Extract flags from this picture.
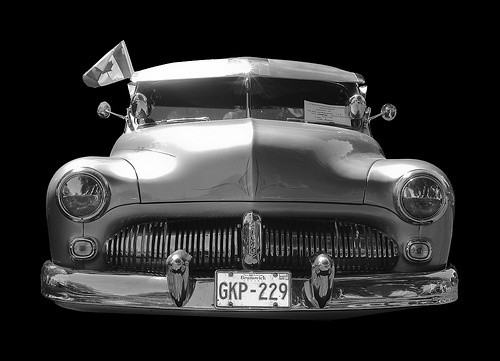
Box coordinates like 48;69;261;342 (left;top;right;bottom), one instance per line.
82;40;135;88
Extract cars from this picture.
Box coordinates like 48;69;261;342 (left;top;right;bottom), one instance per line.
43;54;460;322
